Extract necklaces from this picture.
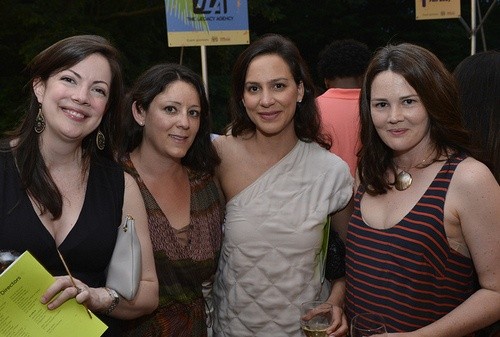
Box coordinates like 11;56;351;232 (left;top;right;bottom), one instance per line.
389;143;437;191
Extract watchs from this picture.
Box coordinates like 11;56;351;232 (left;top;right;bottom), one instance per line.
99;286;119;316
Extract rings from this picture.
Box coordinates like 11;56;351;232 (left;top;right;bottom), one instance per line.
72;285;80;297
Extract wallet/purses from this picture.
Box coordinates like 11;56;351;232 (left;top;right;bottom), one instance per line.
325;230;346;282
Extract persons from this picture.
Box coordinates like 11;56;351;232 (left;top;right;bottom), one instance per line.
202;32;355;337
0;35;161;337
342;42;500;337
452;50;500;187
114;63;226;337
309;39;371;188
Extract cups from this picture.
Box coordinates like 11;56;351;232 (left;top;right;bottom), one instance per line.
350;313;387;337
301;302;333;337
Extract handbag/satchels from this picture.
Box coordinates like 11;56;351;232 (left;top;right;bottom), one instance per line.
106;216;142;301
